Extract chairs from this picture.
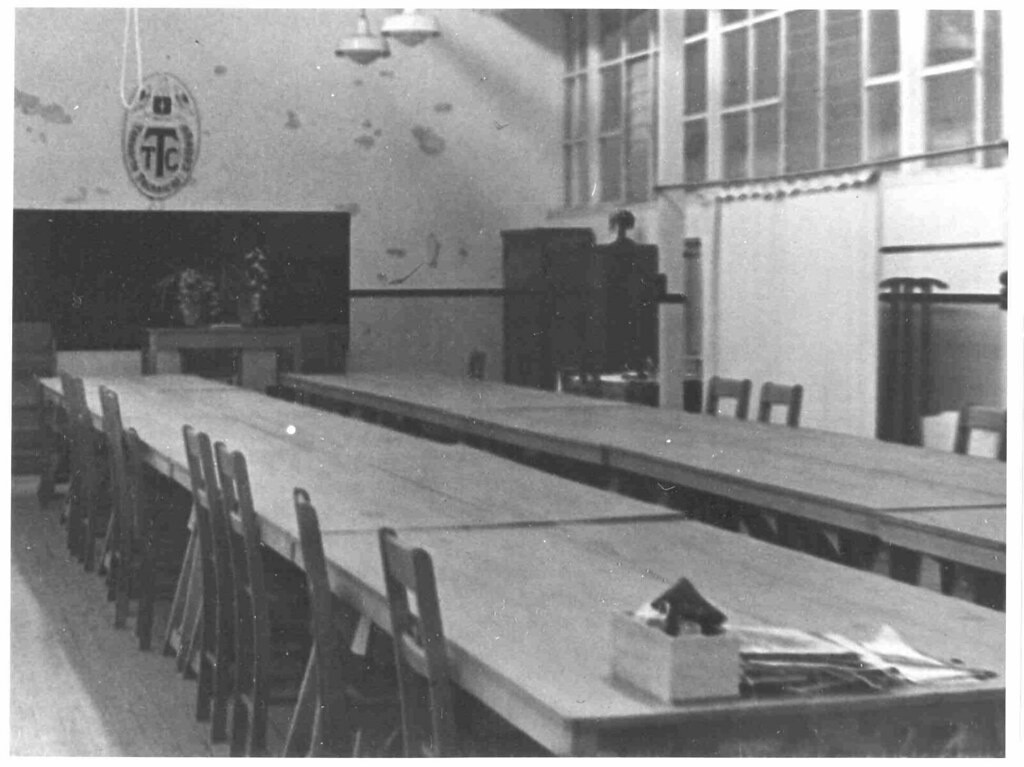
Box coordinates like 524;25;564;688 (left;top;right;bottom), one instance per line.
37;367;555;758
572;373;1007;462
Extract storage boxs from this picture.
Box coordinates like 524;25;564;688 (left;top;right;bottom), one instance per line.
609;609;741;709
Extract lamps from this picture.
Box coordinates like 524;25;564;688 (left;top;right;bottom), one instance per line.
334;9;442;65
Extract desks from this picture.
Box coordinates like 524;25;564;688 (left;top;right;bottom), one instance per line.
38;373;245;434
80;391;450;578
292;517;1006;758
444;407;735;469
604;423;1007;545
278;370;649;431
878;504;1006;595
161;442;684;678
